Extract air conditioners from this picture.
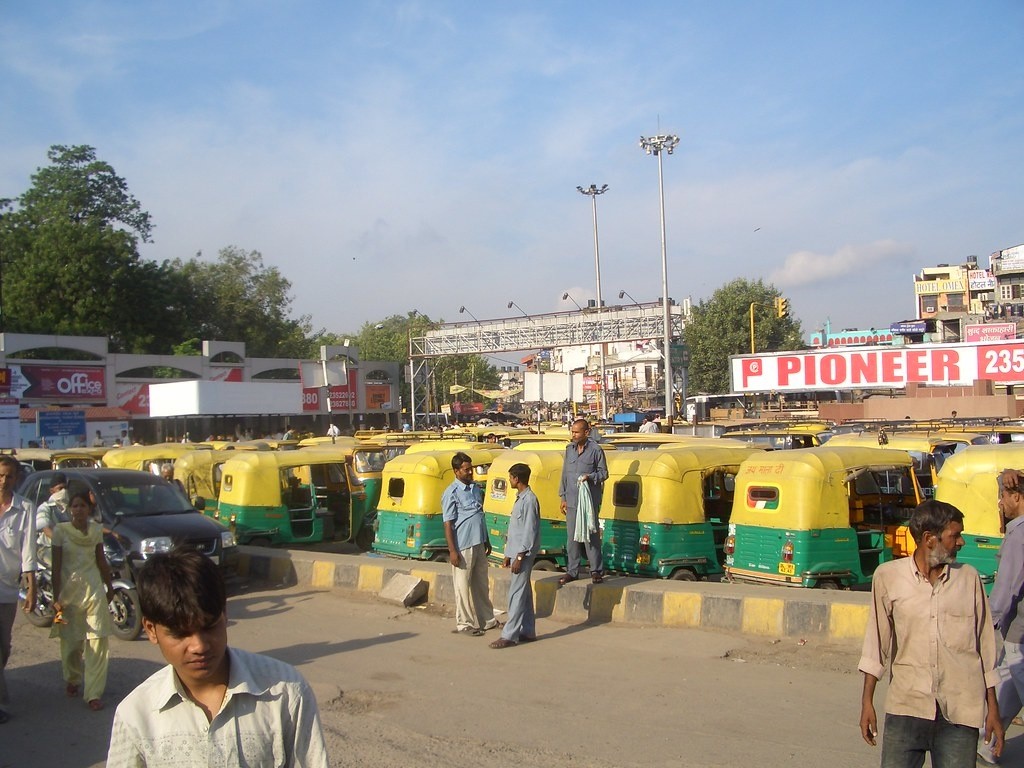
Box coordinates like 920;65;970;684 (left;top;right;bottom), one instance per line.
926;306;935;313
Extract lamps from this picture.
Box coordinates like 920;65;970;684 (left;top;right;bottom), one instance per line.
563;293;589;317
460;307;484;328
508;301;535;324
619;290;643;310
411;309;437;331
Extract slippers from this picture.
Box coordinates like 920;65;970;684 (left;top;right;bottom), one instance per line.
519;634;537;642
491;620;506;628
489;638;514;649
459;628;485;635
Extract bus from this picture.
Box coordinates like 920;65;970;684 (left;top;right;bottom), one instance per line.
678;388;855;422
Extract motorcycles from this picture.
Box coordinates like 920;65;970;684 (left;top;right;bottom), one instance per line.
16;512;144;641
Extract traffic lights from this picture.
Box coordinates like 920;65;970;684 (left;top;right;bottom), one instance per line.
779;298;789;318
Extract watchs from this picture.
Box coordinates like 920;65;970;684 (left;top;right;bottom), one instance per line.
516;554;523;561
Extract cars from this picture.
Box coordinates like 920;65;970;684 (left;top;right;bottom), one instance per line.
16;468;243;596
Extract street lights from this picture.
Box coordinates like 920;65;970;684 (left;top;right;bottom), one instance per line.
639;135;681;416
576;183;610;419
344;339;354;437
318;360;336;444
590;349;652;399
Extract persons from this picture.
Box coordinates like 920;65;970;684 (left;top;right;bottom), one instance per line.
139;462;191;507
441;451;505;636
25;441;48;470
637;416;659;451
370;417;575;434
73;429;144;469
0;472;115;711
559;420;609;584
950;411;957;424
858;499;1006;768
182;423;340;443
104;552;331;768
979;469;1024;767
488;463;540;650
477;433;511;473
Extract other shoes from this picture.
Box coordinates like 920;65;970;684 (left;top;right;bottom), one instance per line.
560;574;579;584
90;699;105;710
592;573;603;583
67;682;79;698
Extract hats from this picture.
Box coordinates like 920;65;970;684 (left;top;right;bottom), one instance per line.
50;472;66;488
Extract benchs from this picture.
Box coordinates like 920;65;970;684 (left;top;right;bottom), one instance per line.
856;529;893;571
288;488;336;518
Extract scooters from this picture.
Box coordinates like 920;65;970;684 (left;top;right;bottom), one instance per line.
483;450;591;572
371;449;506;565
0;441;287;522
596;425;749;451
215;450;367;545
294;445;387;525
934;440;1024;597
720;446;926;589
719;417;1024;499
585;446;767;581
258;427;573;460
